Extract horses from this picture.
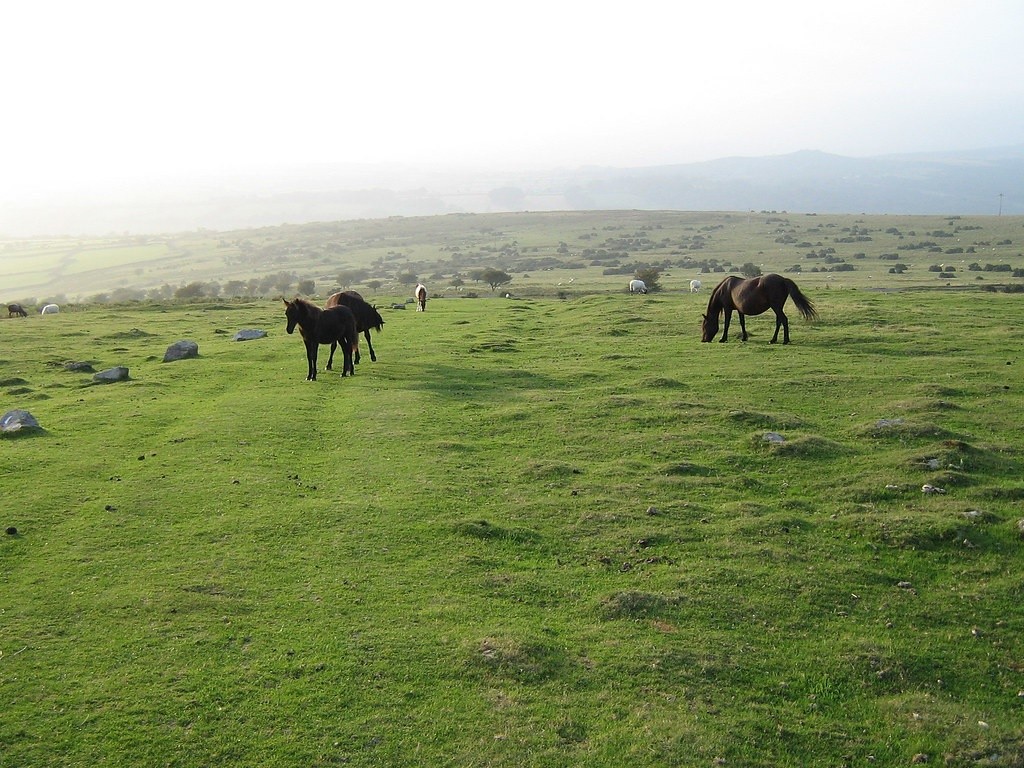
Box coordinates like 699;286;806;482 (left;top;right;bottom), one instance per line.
690;280;702;293
8;304;27;317
324;290;385;373
40;304;59;315
282;297;358;382
629;279;648;295
414;283;428;312
701;273;821;345
